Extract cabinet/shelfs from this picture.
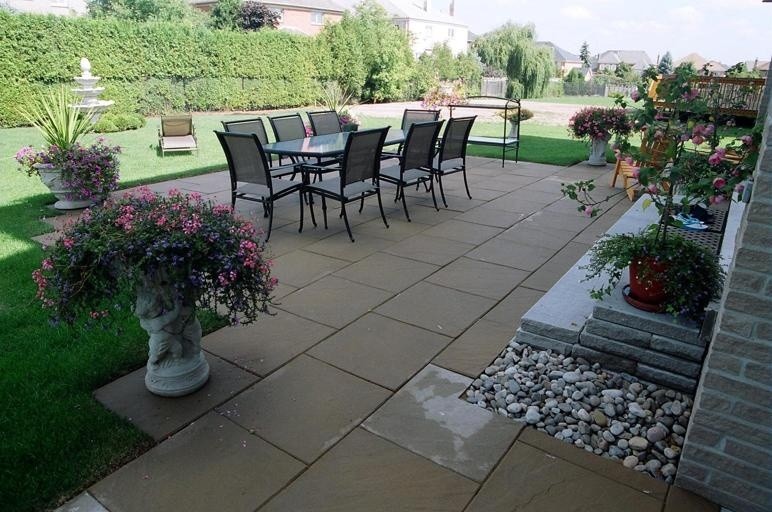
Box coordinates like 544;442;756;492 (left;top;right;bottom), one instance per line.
437;95;522;169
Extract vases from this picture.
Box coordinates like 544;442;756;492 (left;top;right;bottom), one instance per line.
112;258;214;401
583;130;608;169
31;159;107;211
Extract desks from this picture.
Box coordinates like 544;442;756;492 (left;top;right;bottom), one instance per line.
262;127;411;229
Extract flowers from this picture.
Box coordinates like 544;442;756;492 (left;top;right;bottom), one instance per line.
13;137;127;211
29;182;289;350
565;104;632;145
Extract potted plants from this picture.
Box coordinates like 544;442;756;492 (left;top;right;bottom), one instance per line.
574;217;728;323
499;104;536;149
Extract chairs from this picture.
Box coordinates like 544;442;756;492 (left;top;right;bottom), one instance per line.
605;129;682;203
154;114;200;159
220;111;351;206
421;115;478;209
359;119;444;222
306;125;391;243
212;130;307;242
